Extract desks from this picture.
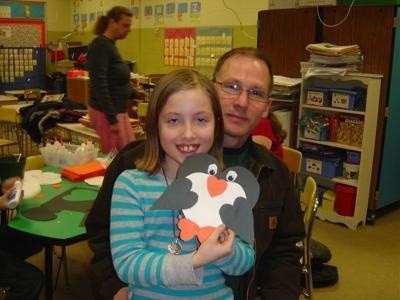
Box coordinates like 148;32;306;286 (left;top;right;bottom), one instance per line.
4;90;48;100
0;95;18;105
7;153;115;300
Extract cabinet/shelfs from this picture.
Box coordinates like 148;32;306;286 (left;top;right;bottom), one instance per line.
296;72;383;231
258;6;322;77
322;7;389;223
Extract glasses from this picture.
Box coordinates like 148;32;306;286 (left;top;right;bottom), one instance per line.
215;79;269;104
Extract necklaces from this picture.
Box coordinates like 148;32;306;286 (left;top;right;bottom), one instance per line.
162;167;182;255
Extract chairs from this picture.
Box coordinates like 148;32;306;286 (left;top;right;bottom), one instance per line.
299;175;316;300
23;154;44;177
278;148;301;188
137;103;148;132
0;108;23;156
25;91;40;104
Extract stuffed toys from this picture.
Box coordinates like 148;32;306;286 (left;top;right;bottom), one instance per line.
149;153;261;248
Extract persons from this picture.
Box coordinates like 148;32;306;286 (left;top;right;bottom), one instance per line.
0;176;47;300
86;7;140;152
110;69;255;300
86;46;308;300
